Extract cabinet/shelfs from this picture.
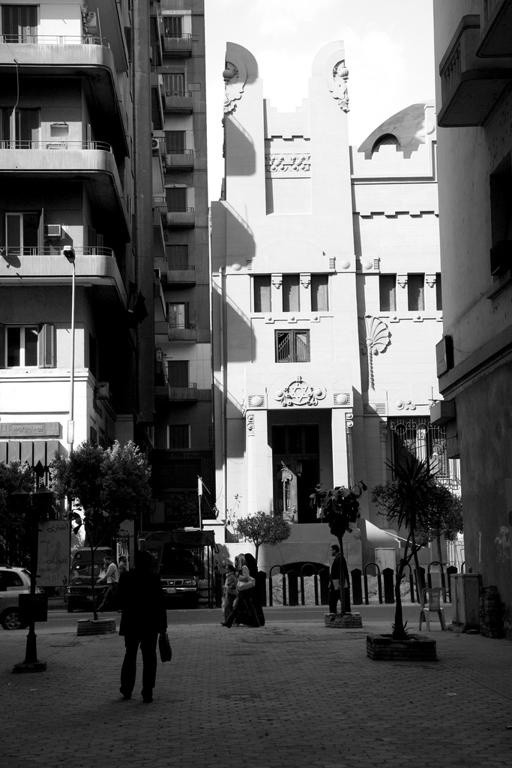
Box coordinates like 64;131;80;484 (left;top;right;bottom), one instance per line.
449;573;480;625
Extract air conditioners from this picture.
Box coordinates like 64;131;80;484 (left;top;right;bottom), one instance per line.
152;138;160;151
44;224;62;238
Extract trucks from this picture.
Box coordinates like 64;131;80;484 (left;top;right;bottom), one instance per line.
145;546;195;609
66;546;115;612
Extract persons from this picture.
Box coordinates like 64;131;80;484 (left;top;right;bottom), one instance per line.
214;554;267;627
118;548;167;704
328;544;349;620
96;554;119;612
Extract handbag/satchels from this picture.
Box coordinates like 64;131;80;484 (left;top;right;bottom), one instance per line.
159;633;172;662
237;575;255;591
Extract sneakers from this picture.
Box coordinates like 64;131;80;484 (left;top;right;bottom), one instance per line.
221;621;232;628
120;687;153;702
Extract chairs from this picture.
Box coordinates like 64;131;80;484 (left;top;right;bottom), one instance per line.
419;587;447;633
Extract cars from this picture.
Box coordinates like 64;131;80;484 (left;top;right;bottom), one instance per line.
0;564;47;629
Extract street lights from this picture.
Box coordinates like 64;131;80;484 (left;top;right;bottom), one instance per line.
62;245;77;602
